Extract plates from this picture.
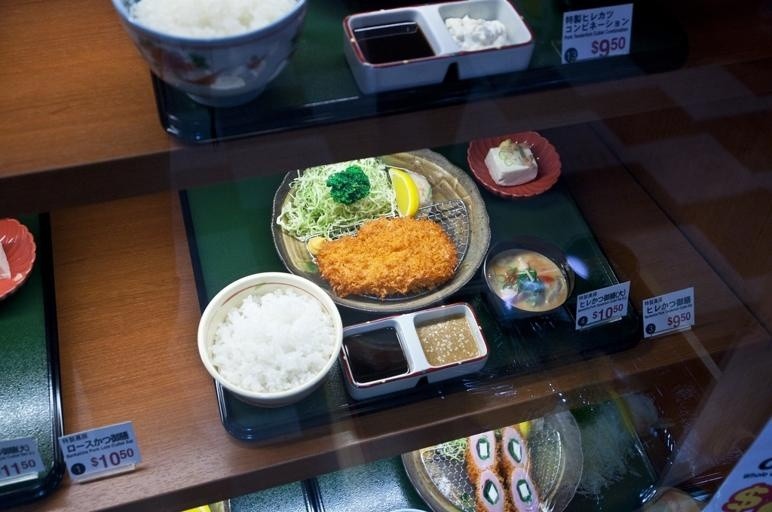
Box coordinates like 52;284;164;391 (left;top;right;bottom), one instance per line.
401;411;584;512
344;1;535;94
342;303;489;399
0;218;39;302
270;148;491;314
467;131;563;199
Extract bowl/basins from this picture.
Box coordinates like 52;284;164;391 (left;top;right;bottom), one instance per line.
114;0;307;109
482;234;576;318
197;271;342;409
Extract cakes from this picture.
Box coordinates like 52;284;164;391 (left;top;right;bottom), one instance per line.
485;138;542;188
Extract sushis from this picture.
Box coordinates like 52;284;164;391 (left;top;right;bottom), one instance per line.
464;426;541;512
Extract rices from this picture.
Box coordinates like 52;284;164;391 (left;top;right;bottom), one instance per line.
209;285;336;395
130;1;303;38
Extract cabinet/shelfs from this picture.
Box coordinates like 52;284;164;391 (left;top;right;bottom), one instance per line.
0;3;771;512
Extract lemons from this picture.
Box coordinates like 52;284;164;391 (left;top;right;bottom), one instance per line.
389;168;416;218
519;421;531;439
